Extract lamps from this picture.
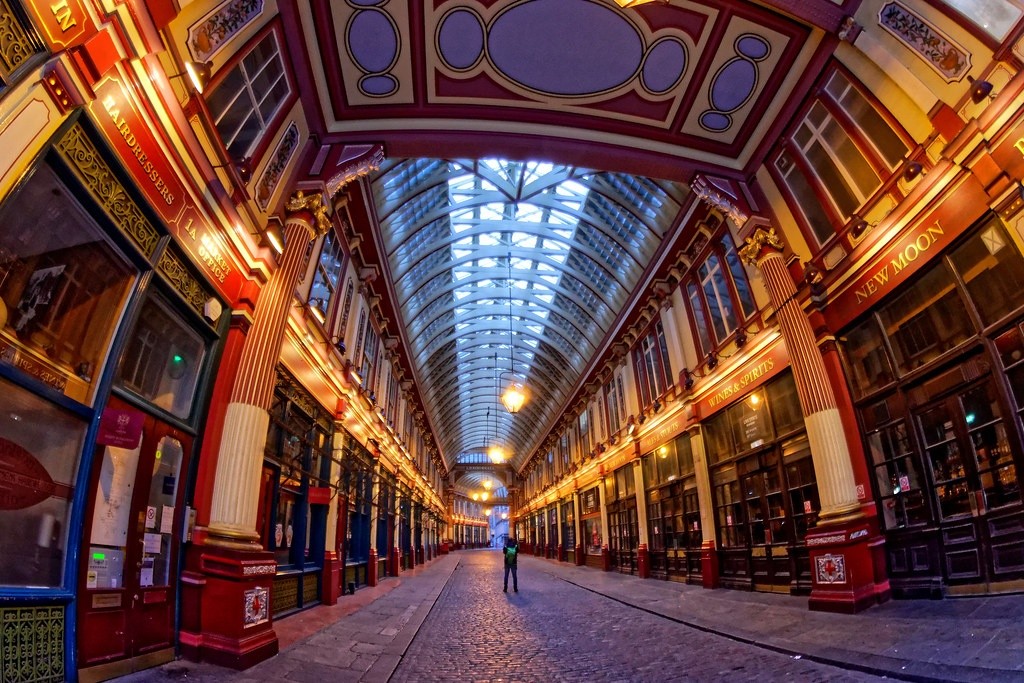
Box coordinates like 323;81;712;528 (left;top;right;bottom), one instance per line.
685;372;706;390
735;326;758;348
967;75;994;105
250;225;285;255
295;296;326;323
627;423;635;435
653;396;673;413
213;157;252;182
499;252;530;414
901;155;925;183
339;364;364;385
638;410;655;424
849;214;875;239
804;261;832;284
708;350;731;369
358;389;447;510
487;352;505;464
320;335;346;355
473;407;627;502
168;60;213;94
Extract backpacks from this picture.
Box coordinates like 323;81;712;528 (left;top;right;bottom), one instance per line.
504;544;518;564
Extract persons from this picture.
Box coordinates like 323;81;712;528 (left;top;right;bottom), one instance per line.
503;537;519;593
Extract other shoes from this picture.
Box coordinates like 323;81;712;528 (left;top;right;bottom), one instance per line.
514;588;518;592
503;590;507;592
